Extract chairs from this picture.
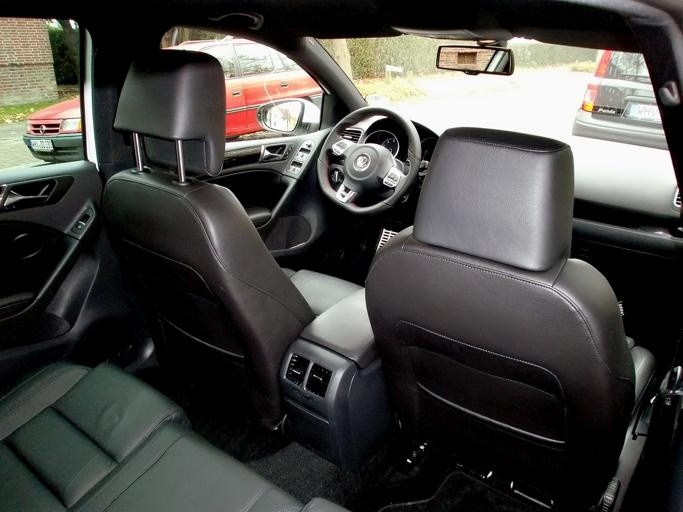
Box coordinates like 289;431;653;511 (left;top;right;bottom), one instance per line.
0;359;194;512
105;435;305;511
301;495;351;512
98;48;364;430
364;127;654;511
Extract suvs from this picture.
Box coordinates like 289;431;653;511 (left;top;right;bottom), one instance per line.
571;49;672;153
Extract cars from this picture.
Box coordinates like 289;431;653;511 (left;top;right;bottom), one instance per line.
19;36;326;163
